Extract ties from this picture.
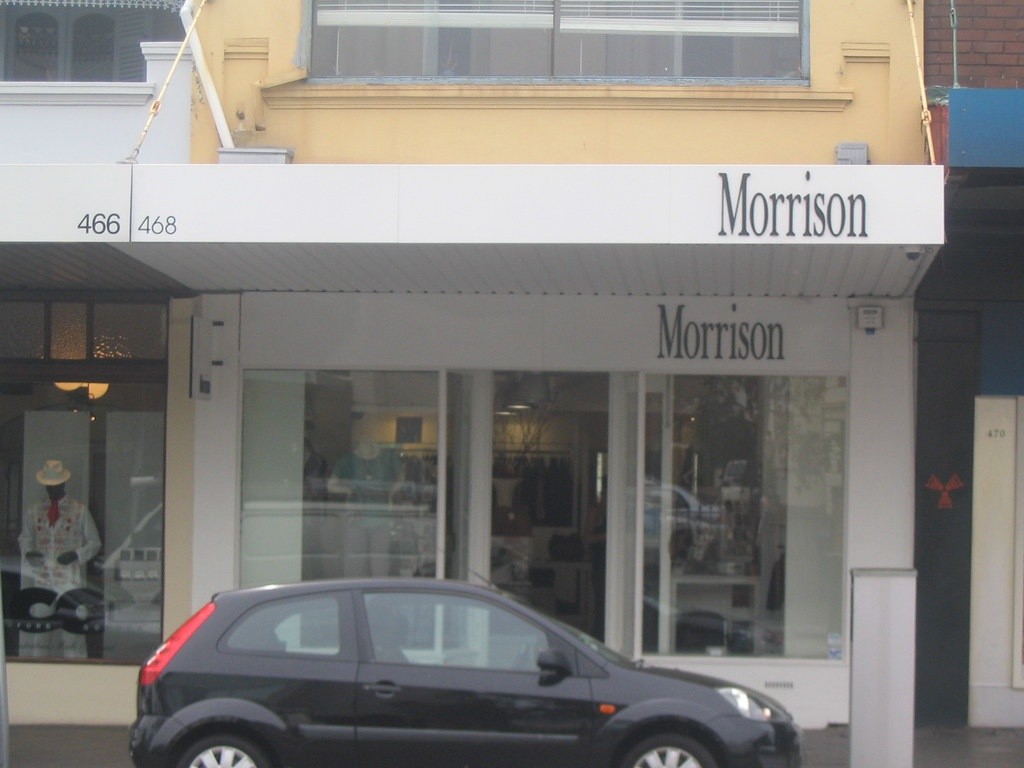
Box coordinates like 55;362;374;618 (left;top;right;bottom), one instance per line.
48;501;60;528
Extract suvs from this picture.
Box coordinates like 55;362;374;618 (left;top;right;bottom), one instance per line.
126;575;804;768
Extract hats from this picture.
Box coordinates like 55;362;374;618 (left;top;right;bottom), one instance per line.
37;460;70;485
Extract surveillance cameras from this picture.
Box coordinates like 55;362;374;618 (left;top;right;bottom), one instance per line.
904;247;921;261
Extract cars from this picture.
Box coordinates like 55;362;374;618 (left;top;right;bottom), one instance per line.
649;484;731;554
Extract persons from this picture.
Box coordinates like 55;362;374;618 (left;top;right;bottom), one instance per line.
19;459;102;658
325;435;407;580
584;474;608;642
